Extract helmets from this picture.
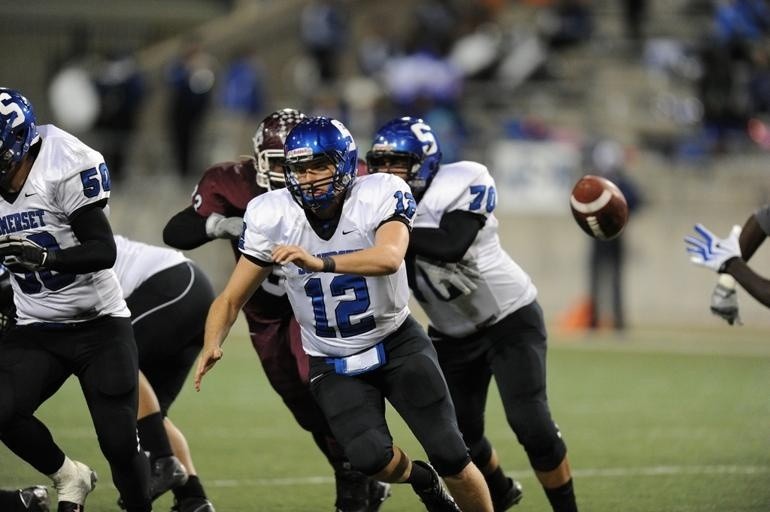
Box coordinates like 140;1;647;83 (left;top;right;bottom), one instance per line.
252;108;303;182
0;87;40;159
283;115;356;204
366;114;443;195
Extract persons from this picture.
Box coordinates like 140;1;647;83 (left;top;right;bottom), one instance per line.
0;85;152;511
111;233;216;511
366;116;579;511
1;1;770;215
585;146;647;329
682;207;770;323
1;485;52;512
164;108;393;511
194;115;496;511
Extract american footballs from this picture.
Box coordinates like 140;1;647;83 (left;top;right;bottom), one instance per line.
570;173;630;241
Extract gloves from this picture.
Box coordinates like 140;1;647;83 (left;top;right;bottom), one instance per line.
206;212;245;238
686;224;741;272
710;277;741;325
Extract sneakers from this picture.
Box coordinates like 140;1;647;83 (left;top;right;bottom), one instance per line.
116;458;216;512
493;477;523;510
0;460;97;512
364;480;391;512
411;455;457;512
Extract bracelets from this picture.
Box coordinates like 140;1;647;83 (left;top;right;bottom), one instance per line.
316;255;336;273
